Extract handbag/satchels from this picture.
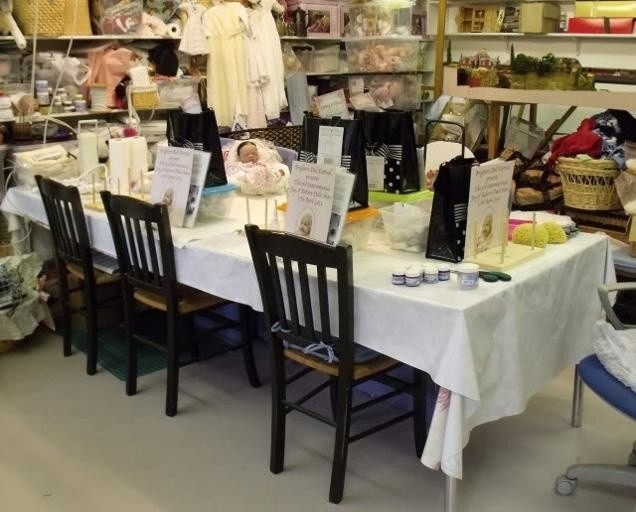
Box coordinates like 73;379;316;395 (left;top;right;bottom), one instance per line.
425;155;515;263
166;107;419;211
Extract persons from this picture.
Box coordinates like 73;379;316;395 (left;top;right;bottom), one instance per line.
476;213;495;252
162;188;174;205
224;141;287;188
297;210;313;236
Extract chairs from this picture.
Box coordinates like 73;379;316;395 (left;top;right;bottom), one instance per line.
244;220;433;504
32;174;128;374
92;186;263;417
554;282;635;503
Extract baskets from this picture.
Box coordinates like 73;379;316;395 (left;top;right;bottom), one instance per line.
292;40;340;73
503;116;545;160
558;156;622;211
131;91;158;110
14;0;93;36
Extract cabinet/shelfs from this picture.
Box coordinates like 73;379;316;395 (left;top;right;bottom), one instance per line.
1;33;222;259
436;0;635;326
235;0;440;148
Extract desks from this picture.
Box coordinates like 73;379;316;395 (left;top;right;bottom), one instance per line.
0;139;611;512
